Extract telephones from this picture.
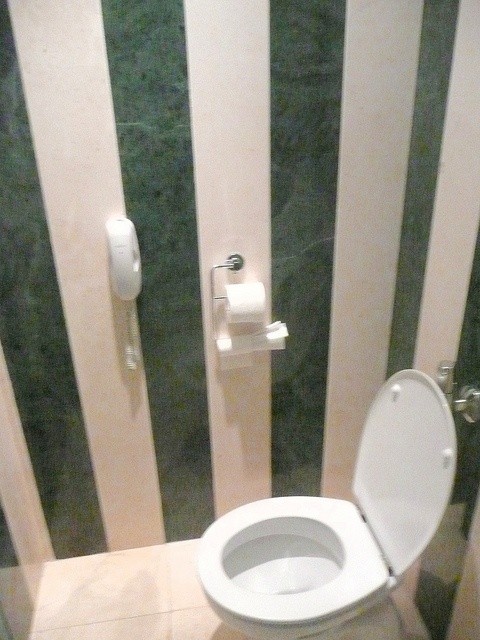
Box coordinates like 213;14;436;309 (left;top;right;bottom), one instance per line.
106;216;143;371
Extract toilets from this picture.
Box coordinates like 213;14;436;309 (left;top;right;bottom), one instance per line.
194;369;457;638
